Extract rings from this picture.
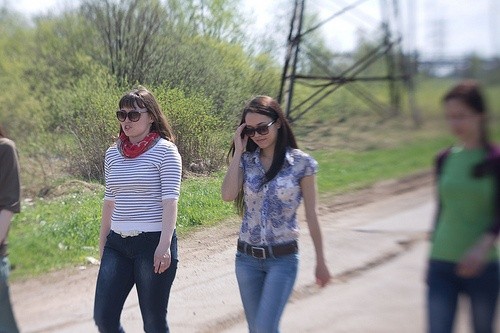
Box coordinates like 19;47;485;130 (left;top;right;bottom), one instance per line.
160;262;165;264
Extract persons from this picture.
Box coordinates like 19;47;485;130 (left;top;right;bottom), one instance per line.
93;89;182;333
425;82;500;332
0;130;22;332
220;94;332;333
